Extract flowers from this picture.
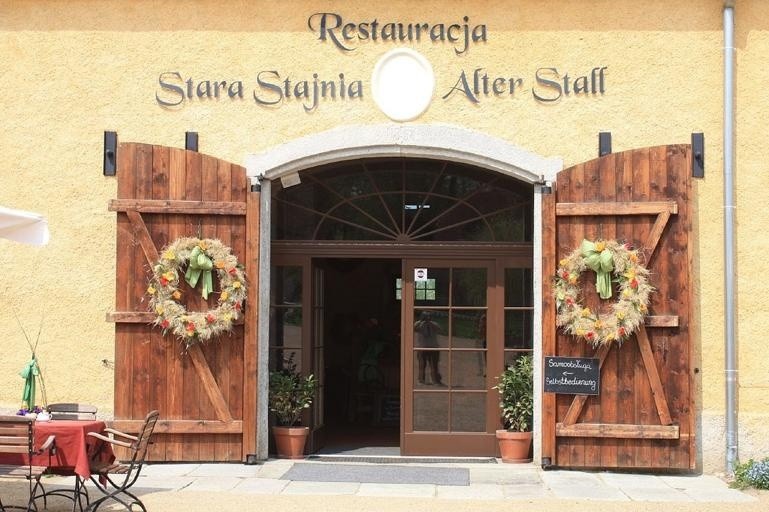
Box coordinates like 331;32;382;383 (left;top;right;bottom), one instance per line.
549;237;654;348
146;235;247;345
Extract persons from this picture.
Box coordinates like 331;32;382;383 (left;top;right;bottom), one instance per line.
413;311;444;386
479;312;486;376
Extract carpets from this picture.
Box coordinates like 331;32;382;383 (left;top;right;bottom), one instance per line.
280;458;471;490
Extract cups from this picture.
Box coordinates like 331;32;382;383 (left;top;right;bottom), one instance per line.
36;413;53;420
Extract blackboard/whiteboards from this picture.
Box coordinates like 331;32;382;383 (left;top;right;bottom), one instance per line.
544;356;600;395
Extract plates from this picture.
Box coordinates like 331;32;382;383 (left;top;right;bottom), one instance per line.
36;419;49;422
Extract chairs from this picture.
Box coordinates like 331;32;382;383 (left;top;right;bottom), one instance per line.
0;402;166;511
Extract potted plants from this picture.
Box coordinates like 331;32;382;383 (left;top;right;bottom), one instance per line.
265;346;321;459
489;353;533;463
